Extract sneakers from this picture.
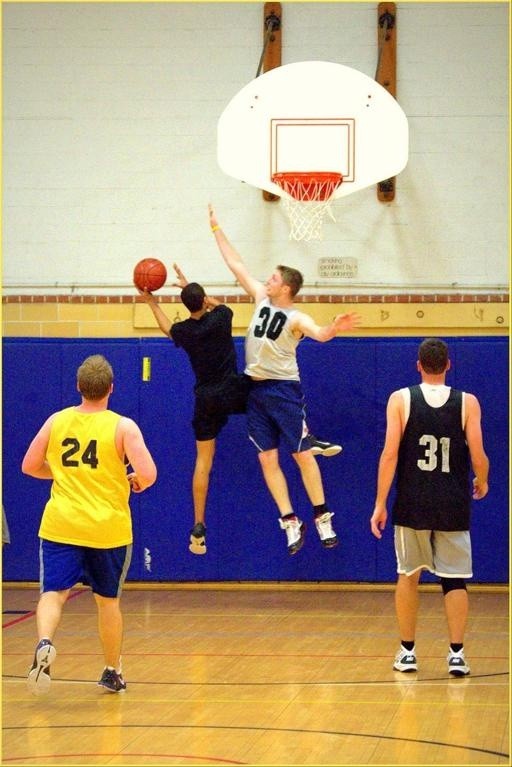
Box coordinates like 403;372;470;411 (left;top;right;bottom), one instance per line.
188;523;207;554
393;643;417;670
27;639;57;695
98;666;127;692
278;515;306;554
309;440;341;456
313;512;338;549
447;646;470;675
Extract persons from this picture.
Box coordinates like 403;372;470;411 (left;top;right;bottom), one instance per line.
207;203;363;555
133;263;342;554
371;338;489;677
21;355;156;693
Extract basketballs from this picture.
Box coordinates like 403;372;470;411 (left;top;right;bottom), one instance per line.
132;257;167;291
